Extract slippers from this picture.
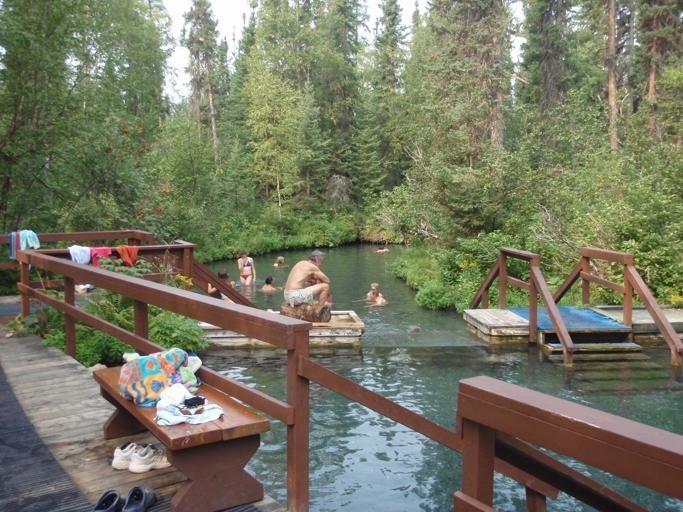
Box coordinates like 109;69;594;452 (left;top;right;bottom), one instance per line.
92;489;125;512
121;485;156;512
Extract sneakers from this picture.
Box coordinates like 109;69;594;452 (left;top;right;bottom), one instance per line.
128;446;171;473
111;442;146;470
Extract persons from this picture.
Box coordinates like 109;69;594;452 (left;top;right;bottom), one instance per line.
273;256;283;266
235;252;257;287
366;282;382;297
349;290;386;308
282;249;331;305
375;242;390;255
261;275;276;291
206;269;233;300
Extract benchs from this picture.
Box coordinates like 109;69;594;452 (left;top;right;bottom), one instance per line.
92;364;273;511
